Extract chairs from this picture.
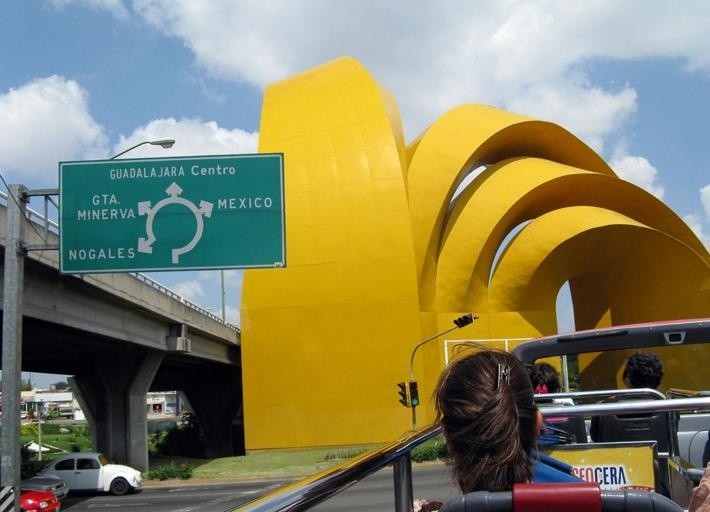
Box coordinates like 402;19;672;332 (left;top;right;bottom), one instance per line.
533;391;678;459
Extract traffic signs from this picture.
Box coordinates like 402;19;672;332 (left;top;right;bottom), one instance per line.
60;153;287;272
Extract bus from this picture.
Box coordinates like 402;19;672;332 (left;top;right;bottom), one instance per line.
236;315;710;512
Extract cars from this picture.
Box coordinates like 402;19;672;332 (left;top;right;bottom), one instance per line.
37;449;144;496
20;464;69;500
19;487;63;512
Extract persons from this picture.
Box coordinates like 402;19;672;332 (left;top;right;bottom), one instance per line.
528;361;589;448
589;351;680;452
436;339;543;491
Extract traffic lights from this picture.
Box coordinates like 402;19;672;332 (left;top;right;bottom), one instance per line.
396;381;407;405
452;313;473;328
409;379;420;406
